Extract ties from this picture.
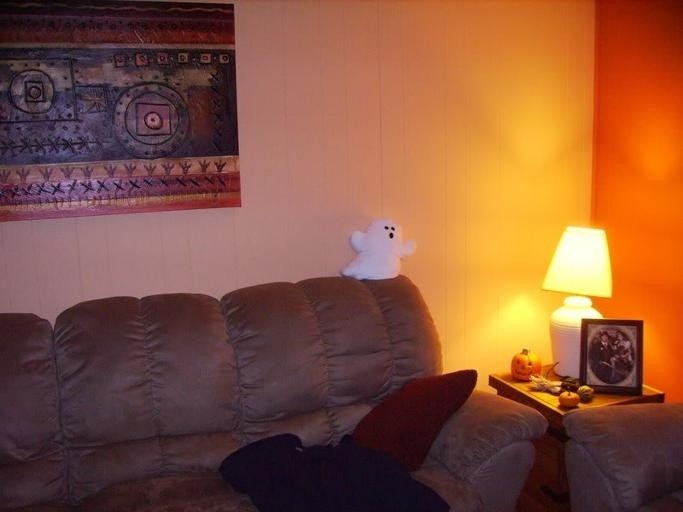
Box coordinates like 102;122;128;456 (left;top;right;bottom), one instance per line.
604;343;607;350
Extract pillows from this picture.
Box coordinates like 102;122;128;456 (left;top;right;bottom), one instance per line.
348;366;479;472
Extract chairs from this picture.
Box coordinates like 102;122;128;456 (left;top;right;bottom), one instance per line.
558;402;681;512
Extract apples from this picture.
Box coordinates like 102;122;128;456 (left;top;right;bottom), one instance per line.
559;390;580;408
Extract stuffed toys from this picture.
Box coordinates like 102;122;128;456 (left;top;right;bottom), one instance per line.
340;216;417;281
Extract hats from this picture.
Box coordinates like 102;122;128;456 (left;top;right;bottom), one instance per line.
599;330;612;338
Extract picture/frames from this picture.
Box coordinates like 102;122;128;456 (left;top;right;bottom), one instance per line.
578;318;645;398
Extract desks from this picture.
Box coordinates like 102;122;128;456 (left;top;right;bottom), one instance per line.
488;368;665;512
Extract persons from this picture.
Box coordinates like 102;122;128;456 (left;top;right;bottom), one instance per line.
590;329;633;383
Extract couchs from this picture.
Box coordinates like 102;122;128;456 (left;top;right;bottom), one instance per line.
0;276;551;511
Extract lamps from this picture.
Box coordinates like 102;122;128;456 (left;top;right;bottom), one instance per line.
541;224;614;382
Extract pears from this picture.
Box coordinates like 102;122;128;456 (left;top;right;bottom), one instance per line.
577;385;594;401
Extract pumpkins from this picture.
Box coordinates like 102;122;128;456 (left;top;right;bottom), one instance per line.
511;348;541;381
